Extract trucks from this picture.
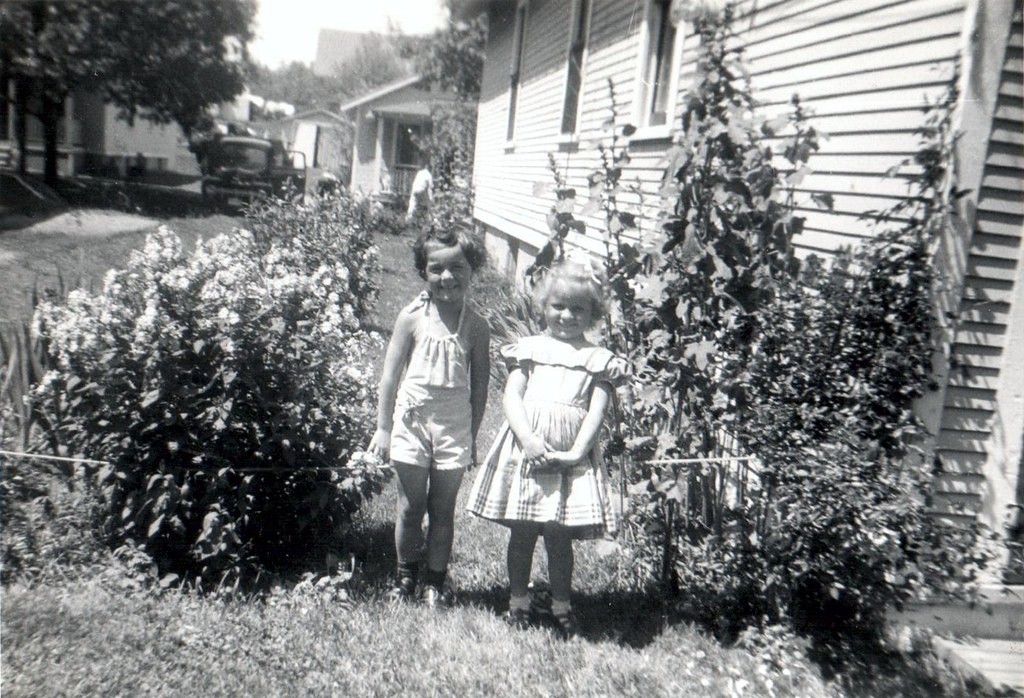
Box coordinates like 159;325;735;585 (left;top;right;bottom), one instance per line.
202;137;307;218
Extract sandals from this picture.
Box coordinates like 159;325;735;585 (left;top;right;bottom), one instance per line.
551;609;585;640
506;608;532;631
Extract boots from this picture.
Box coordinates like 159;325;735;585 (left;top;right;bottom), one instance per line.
383;561;419;601
420;569;445;612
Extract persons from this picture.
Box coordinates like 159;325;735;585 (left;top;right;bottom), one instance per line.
368;228;490;607
465;261;632;640
81;153;147;209
311;172;345;202
